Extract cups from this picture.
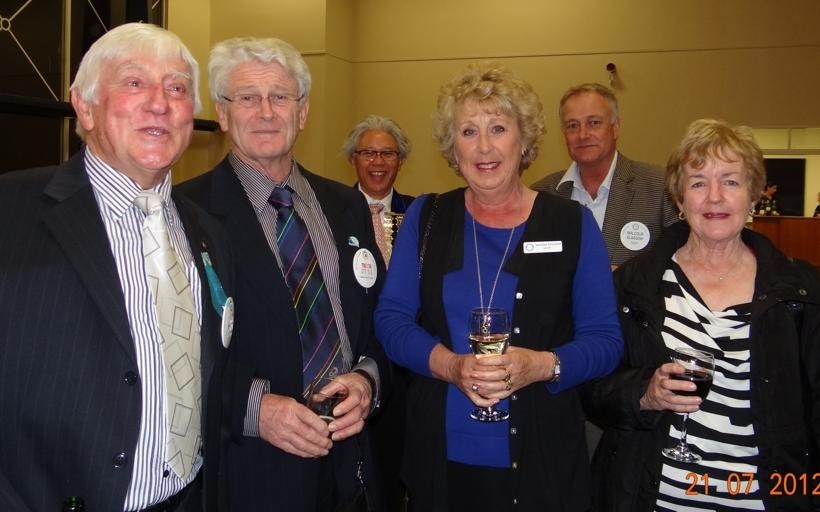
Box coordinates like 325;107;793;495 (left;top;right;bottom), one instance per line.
305;376;350;439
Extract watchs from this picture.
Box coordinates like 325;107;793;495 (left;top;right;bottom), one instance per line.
548;351;561;384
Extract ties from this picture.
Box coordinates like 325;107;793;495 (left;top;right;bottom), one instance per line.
368;204;387;268
266;186;344;415
136;194;201;482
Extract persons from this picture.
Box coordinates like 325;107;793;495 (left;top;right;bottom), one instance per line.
522;81;684;274
365;59;627;509
813;192;820;218
575;118;820;510
337;113;425;278
0;20;240;512
754;182;780;217
167;37;404;510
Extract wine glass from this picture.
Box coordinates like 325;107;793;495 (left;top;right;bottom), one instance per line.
457;308;513;422
661;348;716;464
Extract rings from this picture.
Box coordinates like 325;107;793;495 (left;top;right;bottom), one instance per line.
505;368;511;382
360;416;366;423
506;380;512;390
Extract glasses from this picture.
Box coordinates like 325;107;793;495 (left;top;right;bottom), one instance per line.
354;150;399;161
222;93;307;107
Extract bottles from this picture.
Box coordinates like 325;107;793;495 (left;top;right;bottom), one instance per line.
759;199;776;216
62;496;85;512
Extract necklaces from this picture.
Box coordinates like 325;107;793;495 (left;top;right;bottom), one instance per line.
468;192;521;332
685;242;742;282
